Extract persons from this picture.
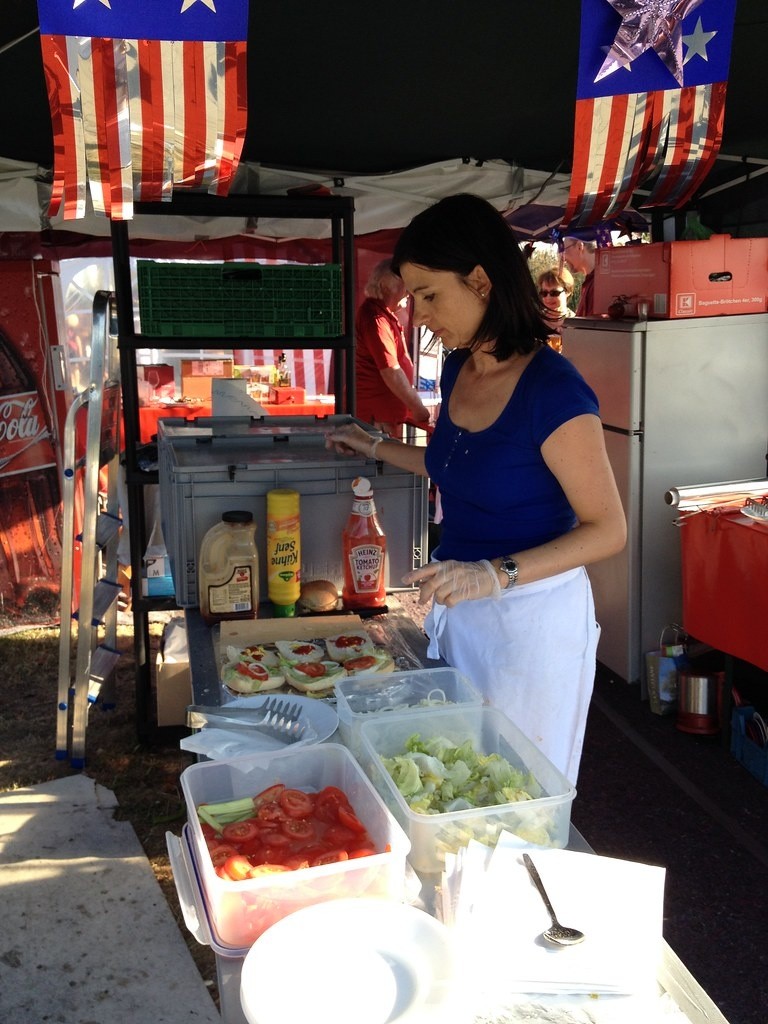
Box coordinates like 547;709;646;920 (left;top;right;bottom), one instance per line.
324;194;627;797
341;258;430;442
562;237;599;317
535;266;576;353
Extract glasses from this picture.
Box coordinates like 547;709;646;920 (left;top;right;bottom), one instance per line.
538;289;567;298
561;242;580;255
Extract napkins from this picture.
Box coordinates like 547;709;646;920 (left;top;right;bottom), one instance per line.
435;830;668;995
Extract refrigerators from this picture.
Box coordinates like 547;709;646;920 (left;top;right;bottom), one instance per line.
560;312;768;702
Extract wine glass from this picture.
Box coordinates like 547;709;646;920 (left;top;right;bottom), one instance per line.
148;370;159;397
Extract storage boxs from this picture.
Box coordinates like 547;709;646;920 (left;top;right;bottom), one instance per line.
156;648;575;955
138;258;341;339
155;414;430;607
595;230;768;320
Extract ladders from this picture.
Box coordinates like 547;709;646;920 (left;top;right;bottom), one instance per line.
55;290;122;769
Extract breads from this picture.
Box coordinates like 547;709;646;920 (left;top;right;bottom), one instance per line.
299;579;338;612
219;627;395;695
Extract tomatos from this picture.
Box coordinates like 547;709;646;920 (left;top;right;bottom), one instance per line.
336;635;366;647
293;644;314;654
294;663;325;677
247;646;261;661
344;656;375;669
239;663;268;682
190;783;378;943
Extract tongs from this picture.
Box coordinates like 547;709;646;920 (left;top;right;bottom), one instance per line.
745;498;768;517
184;698;306;744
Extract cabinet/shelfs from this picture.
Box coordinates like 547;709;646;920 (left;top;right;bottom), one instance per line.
679;505;767;671
109;190;358;753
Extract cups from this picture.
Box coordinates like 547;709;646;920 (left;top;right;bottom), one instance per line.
546;334;562;354
678;670;716;715
638;303;649;322
234;367;269;399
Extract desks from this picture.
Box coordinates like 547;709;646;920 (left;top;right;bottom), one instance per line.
113;390;331;448
185;606;731;1024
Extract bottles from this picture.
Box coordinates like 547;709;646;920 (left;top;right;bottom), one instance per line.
267;490;302;617
341;476;388;610
278;353;292;386
0;328;65;624
199;511;259;624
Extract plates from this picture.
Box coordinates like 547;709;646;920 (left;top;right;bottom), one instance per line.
239;898;471;1023
202;693;340;748
739;505;768;526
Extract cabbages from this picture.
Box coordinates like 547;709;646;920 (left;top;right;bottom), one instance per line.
374;733;553;872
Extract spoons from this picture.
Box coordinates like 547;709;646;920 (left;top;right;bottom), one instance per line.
523;853;586;946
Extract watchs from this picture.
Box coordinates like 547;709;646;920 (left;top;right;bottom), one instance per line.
500;556;518;589
